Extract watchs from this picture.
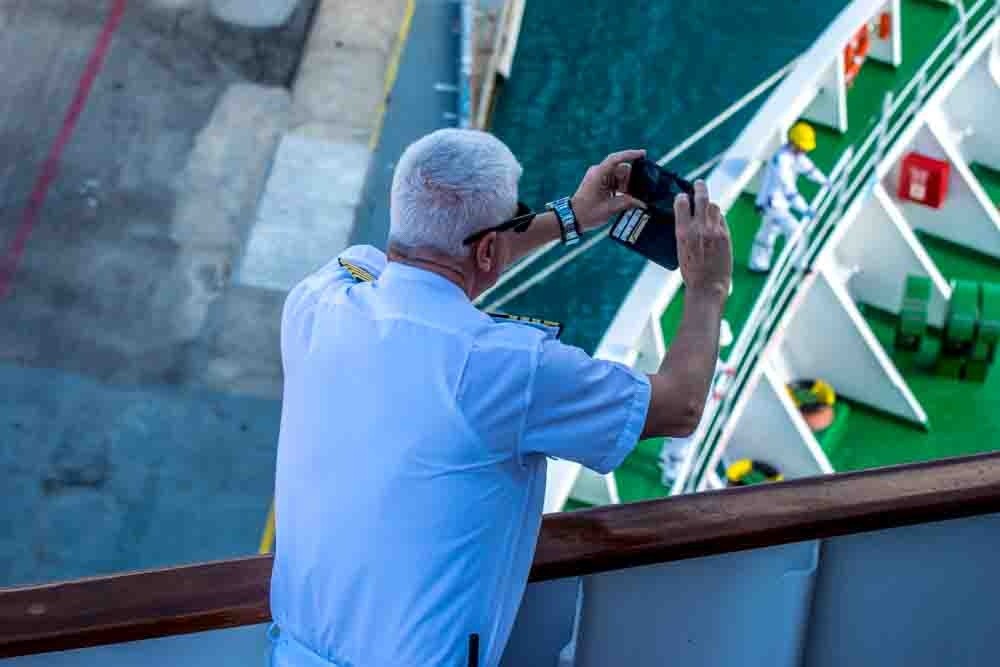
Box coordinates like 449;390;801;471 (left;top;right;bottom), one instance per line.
545;193;583;248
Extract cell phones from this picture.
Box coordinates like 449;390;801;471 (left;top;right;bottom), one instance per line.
628;158;695;218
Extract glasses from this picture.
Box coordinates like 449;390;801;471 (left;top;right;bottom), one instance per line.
463;202;536;246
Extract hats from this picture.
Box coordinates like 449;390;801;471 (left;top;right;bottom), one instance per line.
789;122;817;151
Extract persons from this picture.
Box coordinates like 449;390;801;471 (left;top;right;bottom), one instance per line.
262;125;733;667
745;121;831;275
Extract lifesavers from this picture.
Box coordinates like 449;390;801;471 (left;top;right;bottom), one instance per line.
788;378;835;414
726;458;783;486
844;24;870;84
